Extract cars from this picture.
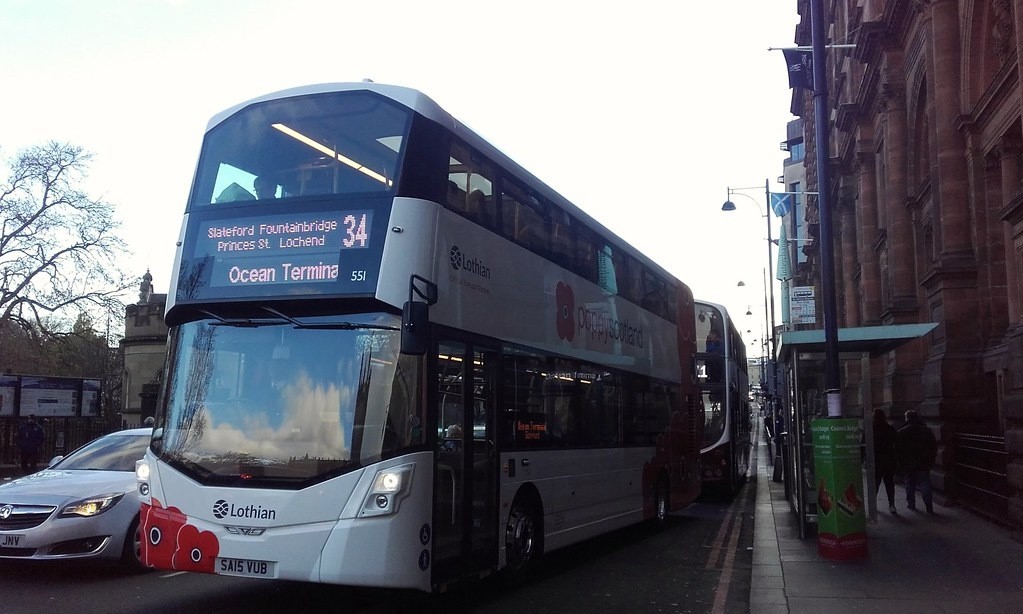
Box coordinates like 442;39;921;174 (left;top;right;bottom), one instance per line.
0;426;152;574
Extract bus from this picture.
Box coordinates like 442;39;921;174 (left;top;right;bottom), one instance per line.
133;84;701;610
694;297;752;502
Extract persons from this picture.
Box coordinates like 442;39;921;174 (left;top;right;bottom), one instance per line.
440;425;464;452
15;414;45;476
445;179;492;225
896;407;939;513
860;407;898;514
252;174;278;200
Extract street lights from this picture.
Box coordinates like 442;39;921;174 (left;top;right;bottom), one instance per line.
726;180;783;484
